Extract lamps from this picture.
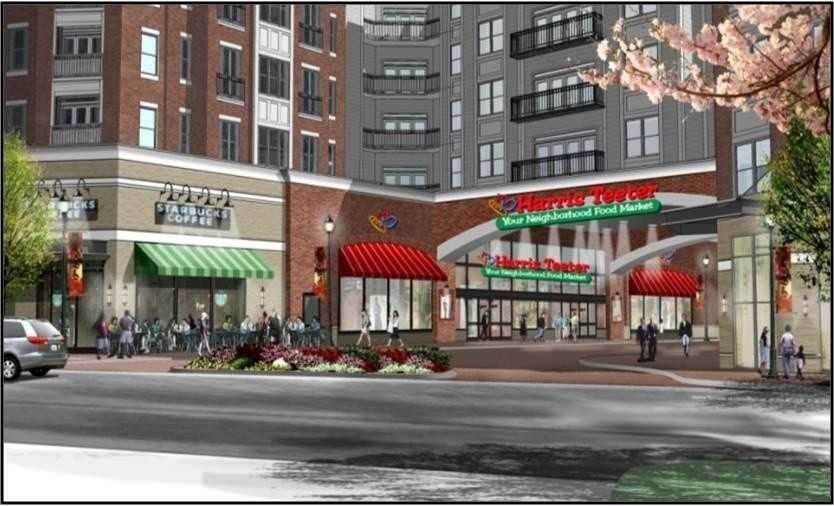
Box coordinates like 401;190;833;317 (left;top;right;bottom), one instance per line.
157;180;235;212
34;177;90;202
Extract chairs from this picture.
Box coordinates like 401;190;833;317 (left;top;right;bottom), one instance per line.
96;325;326;357
